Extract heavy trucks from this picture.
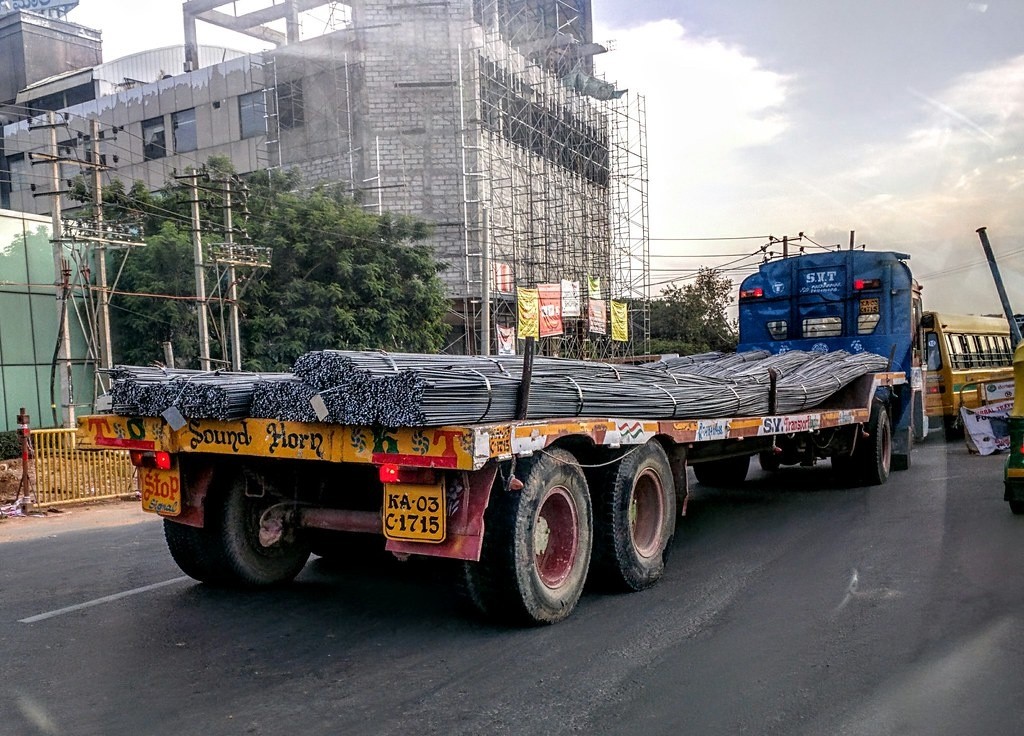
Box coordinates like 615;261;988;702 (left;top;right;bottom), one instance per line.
77;250;925;629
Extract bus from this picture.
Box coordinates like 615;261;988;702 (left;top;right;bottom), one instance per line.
927;311;1015;443
